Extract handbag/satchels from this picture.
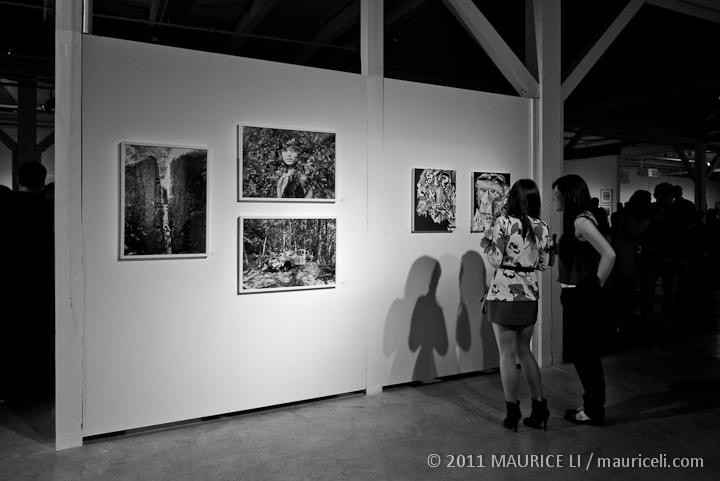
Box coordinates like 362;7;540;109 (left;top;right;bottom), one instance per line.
479;296;488;314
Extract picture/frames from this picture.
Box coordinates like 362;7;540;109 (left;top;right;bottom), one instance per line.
117;123;338;296
599;189;614;217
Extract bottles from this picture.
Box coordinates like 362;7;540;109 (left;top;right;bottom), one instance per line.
548;233;558;266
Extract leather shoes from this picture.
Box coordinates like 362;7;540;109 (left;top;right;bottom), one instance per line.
564;409;605;427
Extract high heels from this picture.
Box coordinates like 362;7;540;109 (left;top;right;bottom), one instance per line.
523;398;550;431
503;399;522;432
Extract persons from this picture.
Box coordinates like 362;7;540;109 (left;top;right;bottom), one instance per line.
591;182;720;301
552;174;616;427
480;178;550;432
277;134;313;198
